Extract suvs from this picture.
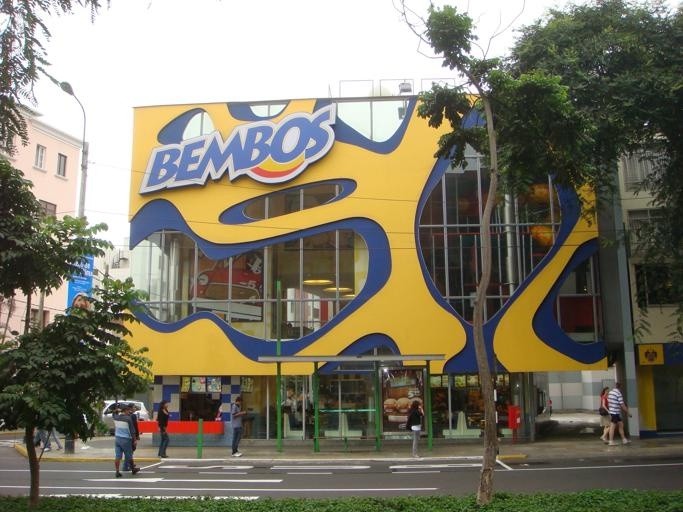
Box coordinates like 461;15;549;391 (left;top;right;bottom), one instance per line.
83;399;149;436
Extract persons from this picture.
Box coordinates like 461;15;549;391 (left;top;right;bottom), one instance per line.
122;401;139;472
404;400;425;459
547;398;552;416
597;386;610;442
110;404;141;478
155;400;174;458
33;424;52;453
229;396;247;458
606;382;632;447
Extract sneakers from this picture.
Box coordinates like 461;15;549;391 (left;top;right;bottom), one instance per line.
114;466;140;479
232;452;243;457
600;436;633;446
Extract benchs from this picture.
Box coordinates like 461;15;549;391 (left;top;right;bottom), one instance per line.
281;411;482;441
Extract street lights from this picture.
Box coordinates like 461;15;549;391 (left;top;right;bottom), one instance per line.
58;76;90;243
